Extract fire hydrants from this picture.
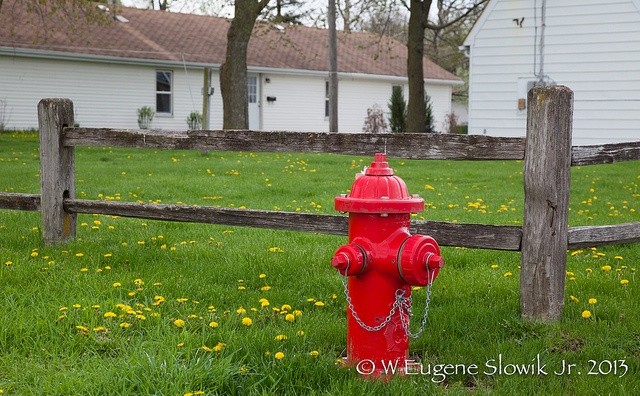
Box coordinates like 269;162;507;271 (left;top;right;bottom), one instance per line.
331;153;444;383
